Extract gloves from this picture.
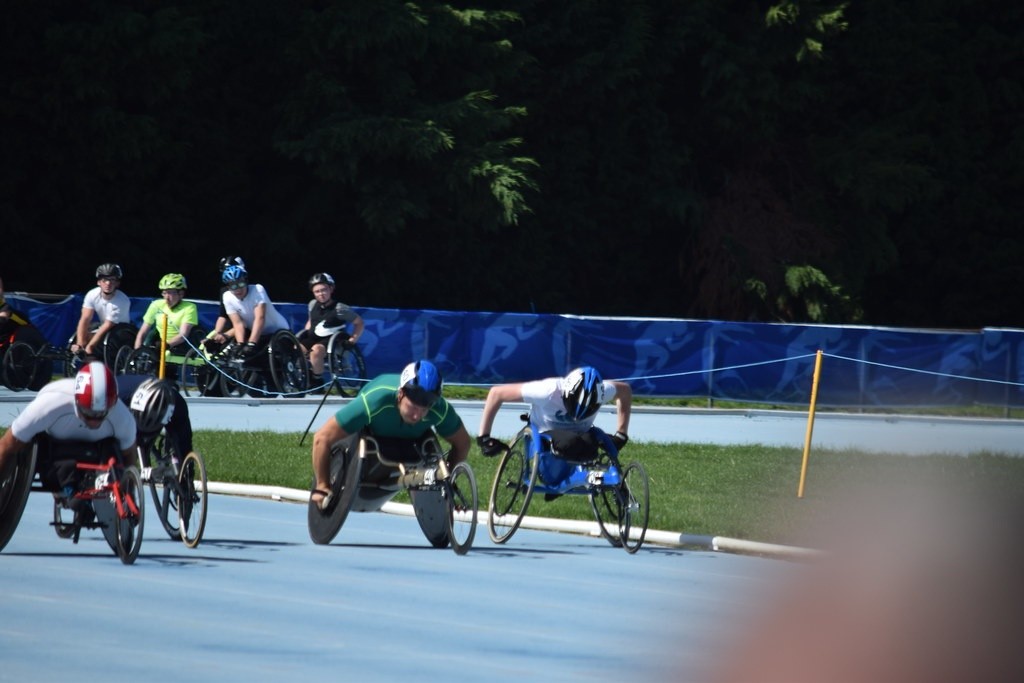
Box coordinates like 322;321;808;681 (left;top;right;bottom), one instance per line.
231;341;258;360
608;432;628;449
477;434;511;457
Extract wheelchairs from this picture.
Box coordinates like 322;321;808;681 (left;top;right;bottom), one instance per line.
53;431;208;549
287;327;367;399
487;412;650;554
0;320;310;398
0;432;144;564
307;426;479;555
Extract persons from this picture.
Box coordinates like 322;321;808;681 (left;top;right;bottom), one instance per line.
297;273;364;395
134;273;198;390
312;361;471;512
0;279;48;361
0;361;195;497
200;256;291;398
71;265;131;373
477;367;632;460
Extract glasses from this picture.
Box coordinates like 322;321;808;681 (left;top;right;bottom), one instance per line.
229;280;246;290
102;277;118;283
77;407;108;421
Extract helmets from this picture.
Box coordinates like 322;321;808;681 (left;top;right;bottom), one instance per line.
310;273;335;288
159;273;187;289
222;265;247;283
130;378;174;431
96;263;122;279
74;361;118;411
400;361;443;408
561;365;604;420
